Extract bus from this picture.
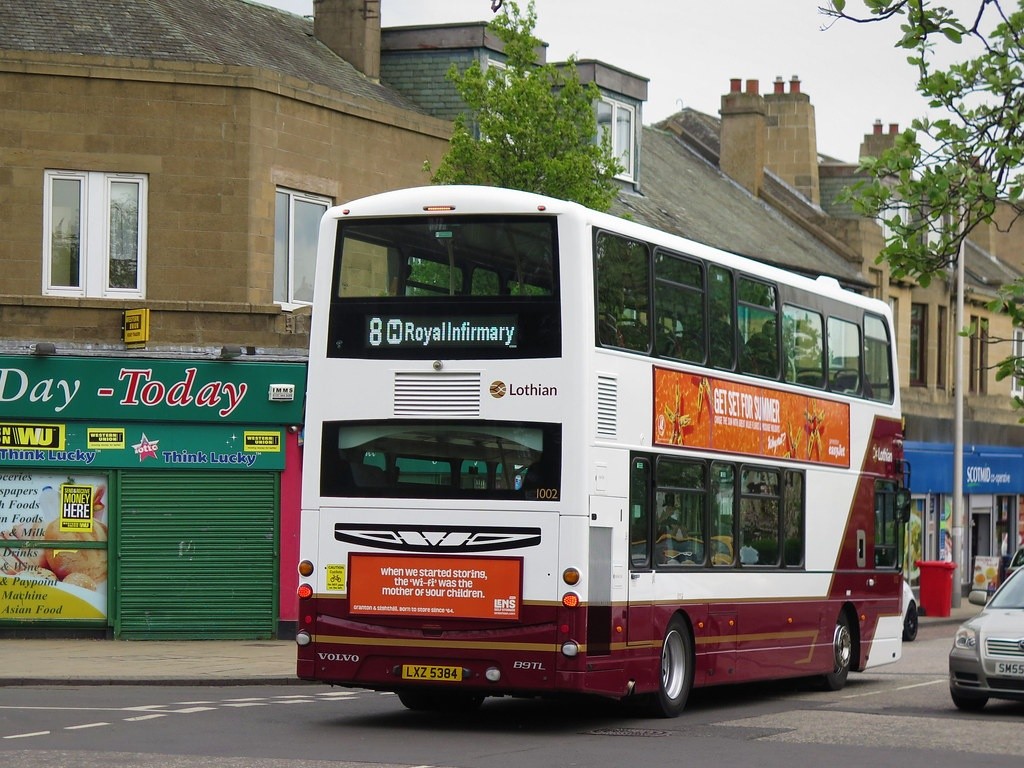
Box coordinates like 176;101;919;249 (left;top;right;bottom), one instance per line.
294;185;914;717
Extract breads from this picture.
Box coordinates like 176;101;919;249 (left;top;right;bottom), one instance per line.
0;515;108;592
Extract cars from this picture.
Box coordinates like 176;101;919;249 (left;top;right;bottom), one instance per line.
1004;546;1024;582
902;579;919;641
948;564;1024;710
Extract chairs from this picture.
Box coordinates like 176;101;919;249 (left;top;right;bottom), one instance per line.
596;312;870;397
633;533;746;566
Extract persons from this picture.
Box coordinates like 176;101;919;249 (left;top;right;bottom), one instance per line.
696;300;745;369
746;320;778;373
